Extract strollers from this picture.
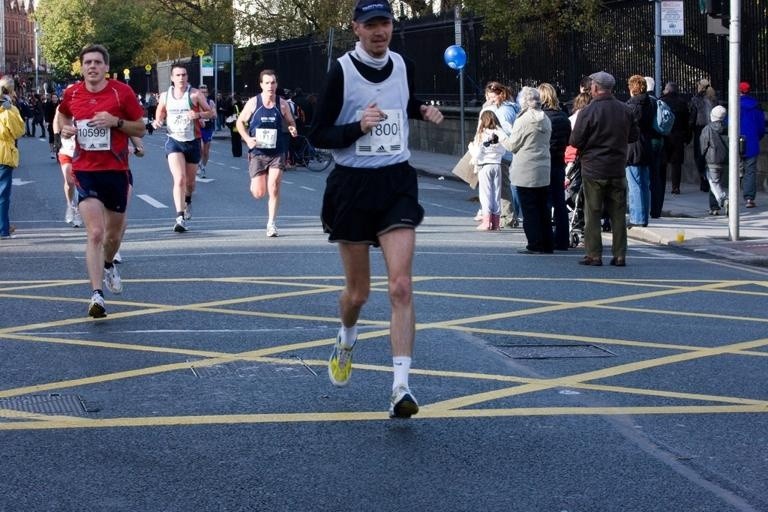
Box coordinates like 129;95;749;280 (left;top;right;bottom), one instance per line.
562;152;610;246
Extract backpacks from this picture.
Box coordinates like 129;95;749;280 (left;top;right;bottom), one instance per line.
649;94;675;136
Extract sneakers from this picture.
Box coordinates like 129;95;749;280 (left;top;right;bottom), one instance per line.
473;176;756;266
0;162;280;263
104;264;122;294
89;291;107;318
328;328;357;386
389;385;418;419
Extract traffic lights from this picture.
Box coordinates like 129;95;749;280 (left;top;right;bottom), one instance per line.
699;0;722;15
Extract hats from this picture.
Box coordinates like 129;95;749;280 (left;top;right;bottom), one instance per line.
589;72;615;88
740;82;749;92
710;106;725;122
354;1;393;23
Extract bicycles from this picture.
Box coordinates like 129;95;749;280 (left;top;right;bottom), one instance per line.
50;126;62;163
294;128;331;172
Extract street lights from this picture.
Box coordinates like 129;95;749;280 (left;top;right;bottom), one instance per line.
32;21;41;95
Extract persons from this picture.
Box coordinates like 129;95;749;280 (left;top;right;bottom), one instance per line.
58;44;147;318
112;135;144;264
472;72;768;266
306;0;446;419
236;70;299;237
0;75;319;237
151;62;215;233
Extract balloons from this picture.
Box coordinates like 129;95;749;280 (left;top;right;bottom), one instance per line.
444;45;466;71
